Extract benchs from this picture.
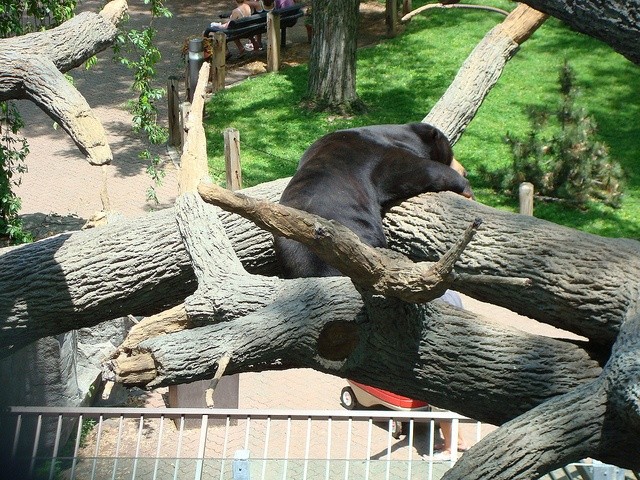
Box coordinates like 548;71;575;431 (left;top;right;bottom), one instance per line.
205;3;304;53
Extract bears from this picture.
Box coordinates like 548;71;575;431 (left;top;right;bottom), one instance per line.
270;122;473;280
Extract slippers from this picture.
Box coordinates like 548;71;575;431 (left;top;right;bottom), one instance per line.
236;48;247;59
225;53;232;60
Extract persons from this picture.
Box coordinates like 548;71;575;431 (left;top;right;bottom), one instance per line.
211;0;252;61
274;1;295;9
243;0;277;50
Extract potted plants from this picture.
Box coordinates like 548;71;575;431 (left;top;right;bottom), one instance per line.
304;8;314;42
